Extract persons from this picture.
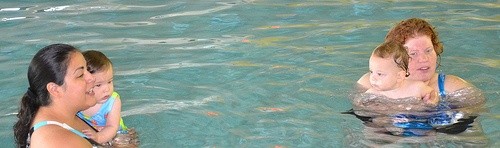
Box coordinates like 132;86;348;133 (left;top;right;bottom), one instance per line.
75;50;140;148
12;44;141;148
356;17;470;95
362;39;431;100
